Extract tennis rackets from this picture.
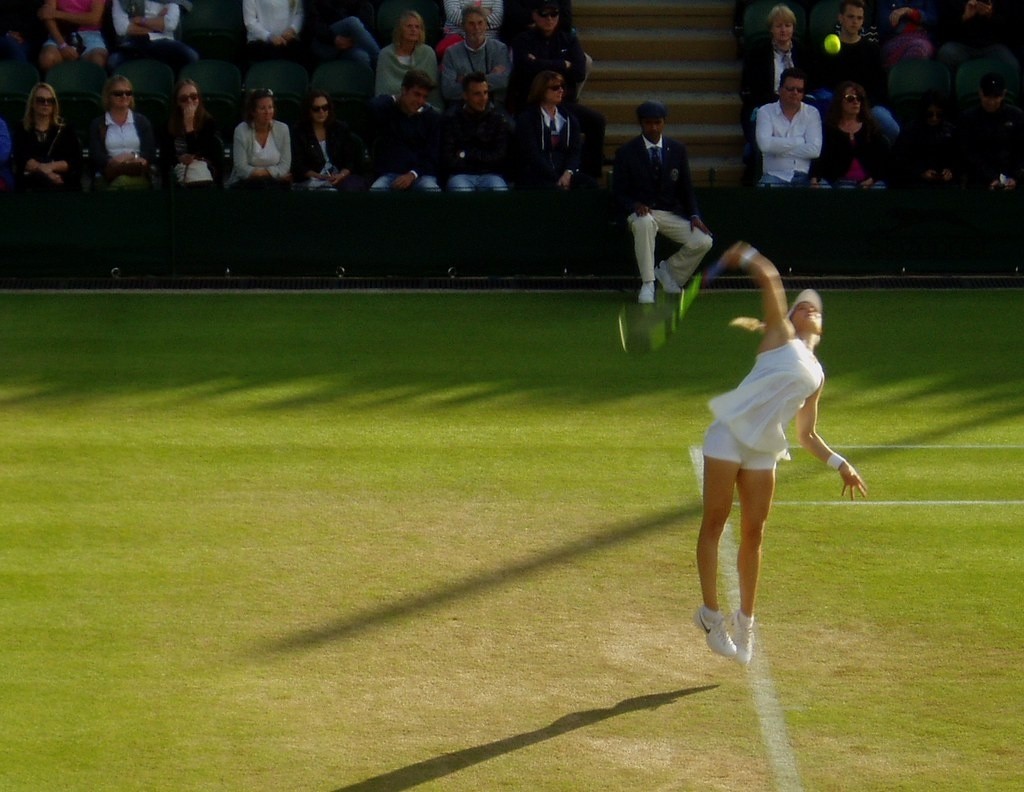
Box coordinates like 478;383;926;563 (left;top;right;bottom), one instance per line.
619;258;725;353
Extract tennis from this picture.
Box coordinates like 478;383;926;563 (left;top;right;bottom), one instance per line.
824;34;841;55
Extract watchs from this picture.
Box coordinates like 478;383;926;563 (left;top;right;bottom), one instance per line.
132;152;138;160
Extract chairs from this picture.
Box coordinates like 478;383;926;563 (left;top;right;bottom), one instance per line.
1;0;445;124
732;0;1024;114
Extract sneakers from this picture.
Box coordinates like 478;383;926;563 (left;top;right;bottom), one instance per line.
638;277;656;304
654;261;681;294
731;612;753;664
694;603;737;657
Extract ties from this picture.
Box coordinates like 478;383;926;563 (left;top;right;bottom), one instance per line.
651;146;662;174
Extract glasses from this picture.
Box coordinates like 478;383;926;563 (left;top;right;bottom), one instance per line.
927;111;943;119
843;94;860;101
175;93;198;102
109;90;131;96
34;96;54;104
535;10;558;18
782;87;804;93
312;103;328;113
547;85;566;91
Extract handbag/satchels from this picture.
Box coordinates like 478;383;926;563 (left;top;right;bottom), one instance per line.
105;158;149;181
175;155;213;184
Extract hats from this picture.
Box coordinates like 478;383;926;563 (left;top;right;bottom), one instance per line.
981;73;1005;96
786;288;824;323
637;102;666;119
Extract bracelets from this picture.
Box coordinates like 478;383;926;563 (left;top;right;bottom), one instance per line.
739;247;757;269
58;42;67;50
140;17;147;26
340;173;346;177
827;451;845;470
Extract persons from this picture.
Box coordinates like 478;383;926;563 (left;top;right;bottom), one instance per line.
612;101;713;303
506;2;606;178
301;0;593;104
290;89;356;188
227;90;293;185
348;68;581;191
0;0;199;69
157;78;225;184
90;73;155;190
0;82;82;190
697;241;868;667
243;0;316;67
731;1;1024;188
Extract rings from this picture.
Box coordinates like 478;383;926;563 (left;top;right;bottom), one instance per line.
288;33;290;35
334;176;336;180
